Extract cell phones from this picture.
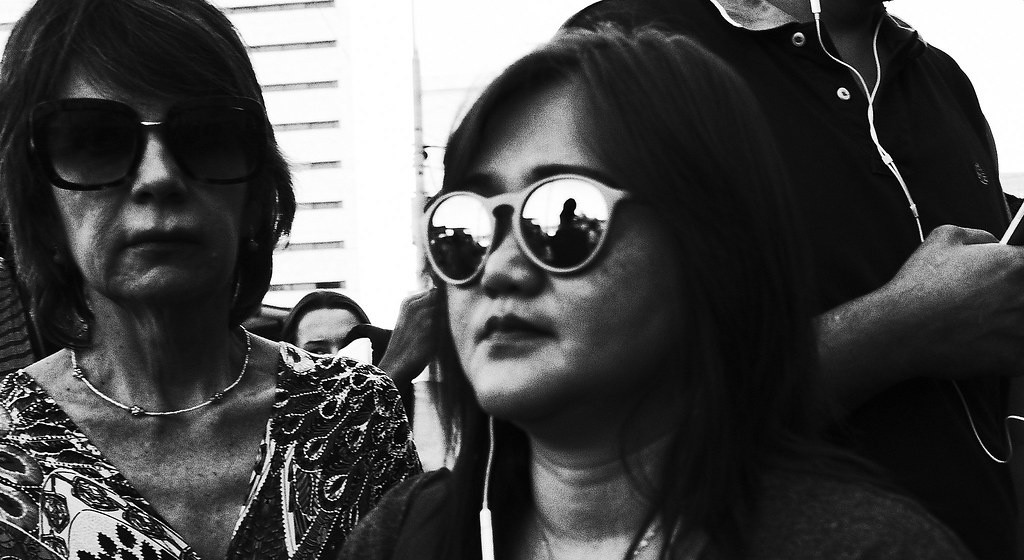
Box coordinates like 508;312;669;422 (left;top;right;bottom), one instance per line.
1000;202;1024;246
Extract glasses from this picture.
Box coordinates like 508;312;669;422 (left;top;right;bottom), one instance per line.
421;172;641;286
26;93;277;187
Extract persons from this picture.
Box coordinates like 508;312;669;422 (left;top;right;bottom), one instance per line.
1;0;1024;560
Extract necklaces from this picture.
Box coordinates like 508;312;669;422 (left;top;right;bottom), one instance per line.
634;517;666;555
72;325;252;414
